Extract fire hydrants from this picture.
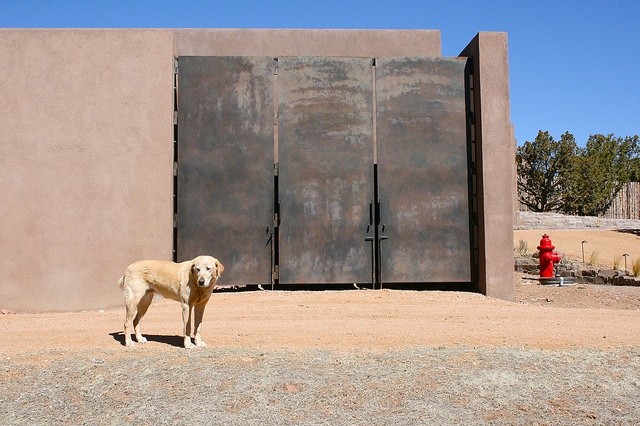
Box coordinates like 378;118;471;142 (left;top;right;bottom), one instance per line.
537;233;561;277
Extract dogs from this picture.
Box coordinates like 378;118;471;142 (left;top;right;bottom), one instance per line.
118;254;225;348
